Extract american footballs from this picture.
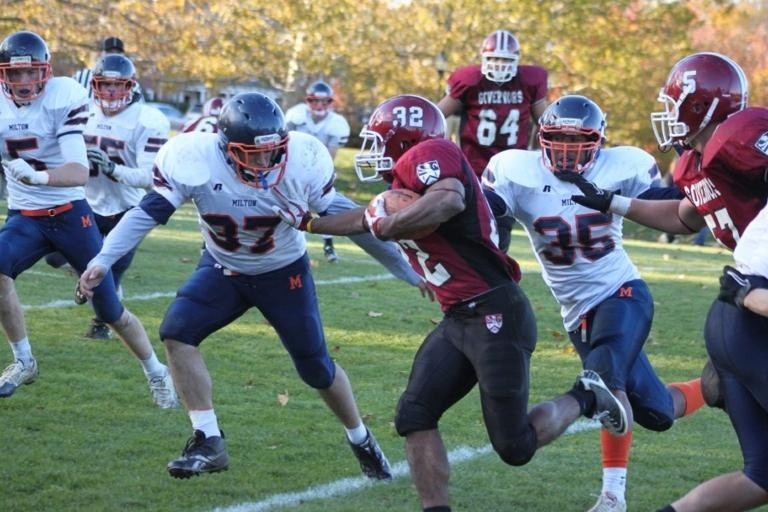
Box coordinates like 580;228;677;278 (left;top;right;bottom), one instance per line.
368;189;440;239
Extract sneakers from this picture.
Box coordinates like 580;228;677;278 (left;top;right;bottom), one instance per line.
585;491;627;512
346;427;394;480
323;246;337;263
576;369;628;436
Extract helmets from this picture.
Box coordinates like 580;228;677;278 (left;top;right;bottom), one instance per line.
0;32;50;101
91;54;136;112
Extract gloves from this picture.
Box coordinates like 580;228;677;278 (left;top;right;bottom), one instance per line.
363;196;389;241
87;147;115;174
717;266;768;312
553;170;613;213
272;178;320;233
1;157;49;185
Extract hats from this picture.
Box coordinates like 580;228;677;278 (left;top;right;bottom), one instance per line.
104;37;123;52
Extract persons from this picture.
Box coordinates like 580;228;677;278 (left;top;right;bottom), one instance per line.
285;86;351;261
559;51;768;408
181;95;228;134
71;36;146;101
436;29;549;258
649;199;768;512
268;92;628;512
45;54;171;342
73;90;438;487
0;31;181;411
480;94;706;511
